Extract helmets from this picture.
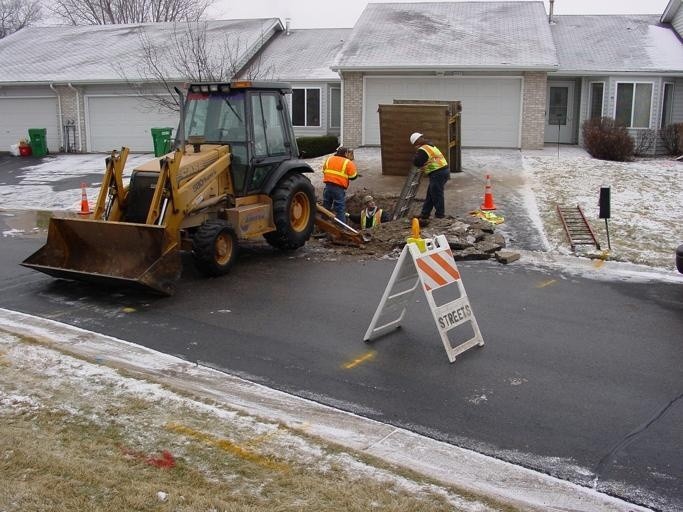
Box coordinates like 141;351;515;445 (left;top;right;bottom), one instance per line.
409;133;423;144
363;195;373;205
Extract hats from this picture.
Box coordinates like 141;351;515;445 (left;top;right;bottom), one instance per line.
337;146;352;155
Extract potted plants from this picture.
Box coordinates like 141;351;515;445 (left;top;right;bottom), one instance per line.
18;138;30;156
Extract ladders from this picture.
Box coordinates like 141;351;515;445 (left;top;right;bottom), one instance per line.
390;165;425;220
557;204;601;250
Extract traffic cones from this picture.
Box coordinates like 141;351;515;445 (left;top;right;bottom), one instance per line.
480;174;500;211
77;180;94;215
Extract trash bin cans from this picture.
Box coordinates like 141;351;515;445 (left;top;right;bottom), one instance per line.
28;128;49;157
151;128;174;157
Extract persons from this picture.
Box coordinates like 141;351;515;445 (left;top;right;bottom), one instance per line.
410;132;450;221
320;146;358;227
345;195;392;231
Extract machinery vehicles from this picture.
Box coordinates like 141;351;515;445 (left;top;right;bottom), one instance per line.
16;76;374;299
